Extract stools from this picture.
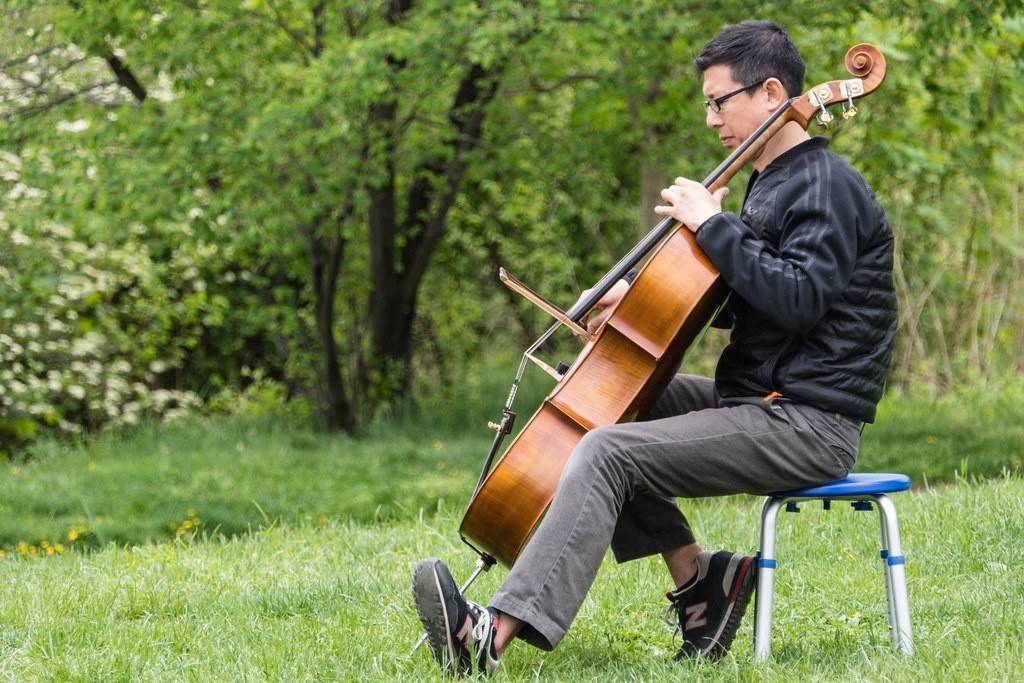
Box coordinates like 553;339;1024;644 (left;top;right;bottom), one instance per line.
752;473;915;661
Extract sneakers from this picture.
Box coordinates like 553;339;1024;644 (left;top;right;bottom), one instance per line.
411;556;500;681
664;550;757;665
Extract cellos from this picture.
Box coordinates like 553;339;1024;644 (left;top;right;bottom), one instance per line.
408;40;887;655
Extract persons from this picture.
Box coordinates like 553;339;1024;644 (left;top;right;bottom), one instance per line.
413;19;898;678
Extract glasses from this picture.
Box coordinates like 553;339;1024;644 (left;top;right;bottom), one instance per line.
701;75;784;113
